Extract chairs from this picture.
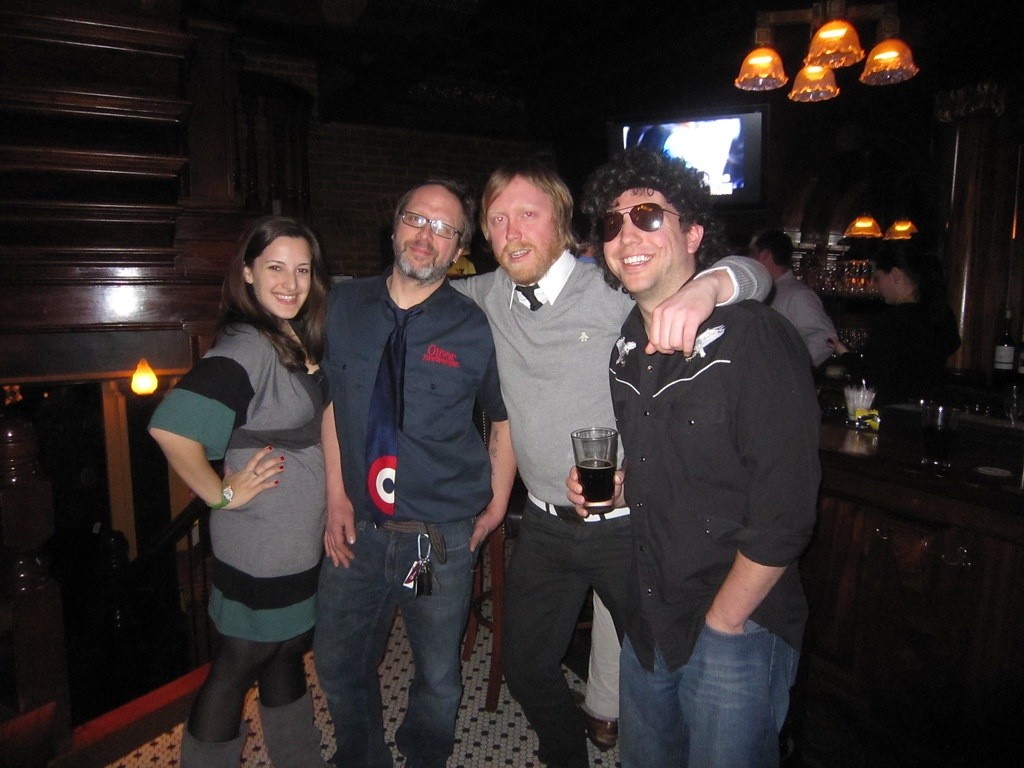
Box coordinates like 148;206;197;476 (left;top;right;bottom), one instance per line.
458;398;594;716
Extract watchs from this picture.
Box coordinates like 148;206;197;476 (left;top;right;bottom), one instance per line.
206;480;233;509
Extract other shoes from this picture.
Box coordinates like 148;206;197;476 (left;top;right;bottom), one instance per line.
571;688;619;753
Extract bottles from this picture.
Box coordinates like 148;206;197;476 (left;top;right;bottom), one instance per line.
831;255;881;294
841;362;880;421
992;306;1024;380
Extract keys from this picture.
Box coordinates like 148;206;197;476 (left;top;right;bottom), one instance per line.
402;532;442;599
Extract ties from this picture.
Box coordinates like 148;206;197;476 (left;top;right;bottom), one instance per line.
365;296;423;527
516;282;542;312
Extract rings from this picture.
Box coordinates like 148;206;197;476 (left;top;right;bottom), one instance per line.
253;468;259;478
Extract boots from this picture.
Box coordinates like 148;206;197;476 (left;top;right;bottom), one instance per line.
258;683;329;768
179;719;248;768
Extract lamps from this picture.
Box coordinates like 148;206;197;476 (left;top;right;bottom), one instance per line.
840;205;920;245
726;0;925;108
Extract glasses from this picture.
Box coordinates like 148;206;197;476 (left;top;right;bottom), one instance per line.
596;203;688;244
401;209;464;240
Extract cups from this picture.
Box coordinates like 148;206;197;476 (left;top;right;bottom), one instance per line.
571;427;618;514
919;404;958;468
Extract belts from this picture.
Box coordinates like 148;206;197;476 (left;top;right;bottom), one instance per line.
528;490;634;524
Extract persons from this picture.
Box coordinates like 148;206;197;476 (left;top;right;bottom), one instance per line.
565;144;821;768
314;172;517;768
446;157;772;768
751;225;847;369
146;214;327;768
824;238;962;404
